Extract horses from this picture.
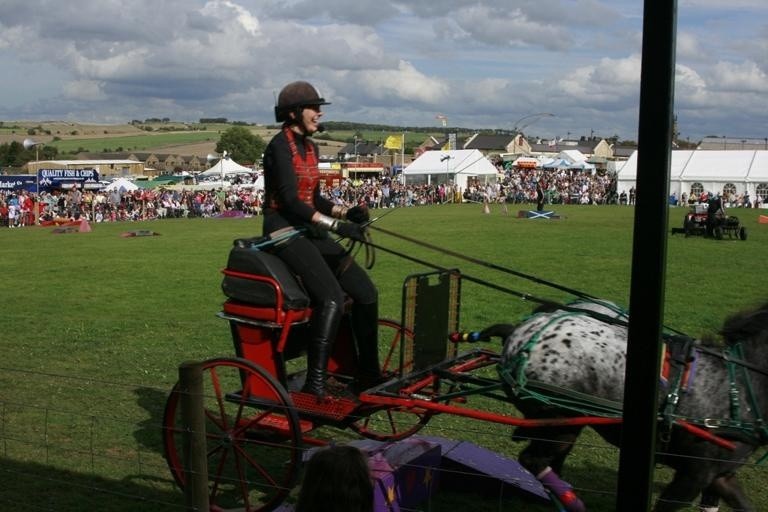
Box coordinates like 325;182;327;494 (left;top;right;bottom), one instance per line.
446;297;768;511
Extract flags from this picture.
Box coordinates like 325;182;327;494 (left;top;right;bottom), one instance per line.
382;133;405;150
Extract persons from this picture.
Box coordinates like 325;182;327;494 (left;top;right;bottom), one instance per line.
320;170;622;212
0;172;265;228
620;185;764;208
262;81;389;402
704;192;730;236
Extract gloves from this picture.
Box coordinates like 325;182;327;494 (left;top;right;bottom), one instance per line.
335;206;369;241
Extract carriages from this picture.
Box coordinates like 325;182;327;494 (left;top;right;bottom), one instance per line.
163;237;768;508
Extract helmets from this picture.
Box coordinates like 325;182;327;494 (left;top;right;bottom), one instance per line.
275;81;331;120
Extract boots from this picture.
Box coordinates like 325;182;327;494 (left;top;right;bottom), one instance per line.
301;299;389;395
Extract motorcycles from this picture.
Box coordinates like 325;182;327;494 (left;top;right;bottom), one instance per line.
714;215;747;240
672;195;722;238
